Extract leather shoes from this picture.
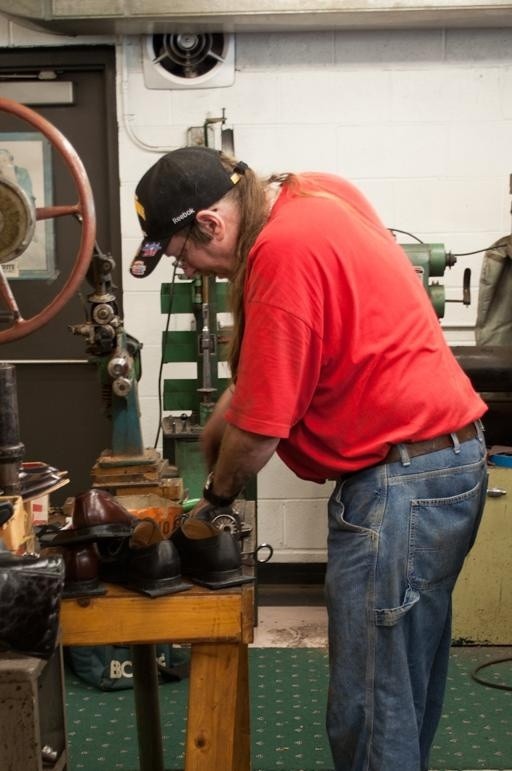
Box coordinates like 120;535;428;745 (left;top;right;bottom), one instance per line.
113;519;182;589
169;514;242;574
65;547;100;592
73;489;132;527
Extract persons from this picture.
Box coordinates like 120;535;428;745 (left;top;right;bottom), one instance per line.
130;147;490;771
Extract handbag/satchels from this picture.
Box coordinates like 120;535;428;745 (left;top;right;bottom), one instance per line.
68;641;191;691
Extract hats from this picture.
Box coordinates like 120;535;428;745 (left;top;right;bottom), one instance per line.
130;146;247;279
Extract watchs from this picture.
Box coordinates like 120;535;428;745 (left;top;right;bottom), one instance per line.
202;470;234;507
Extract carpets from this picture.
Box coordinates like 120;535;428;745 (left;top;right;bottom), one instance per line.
61;645;511;770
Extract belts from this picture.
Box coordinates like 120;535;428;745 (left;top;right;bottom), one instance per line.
382;418;479;465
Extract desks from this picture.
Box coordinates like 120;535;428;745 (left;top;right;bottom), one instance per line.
21;497;259;770
